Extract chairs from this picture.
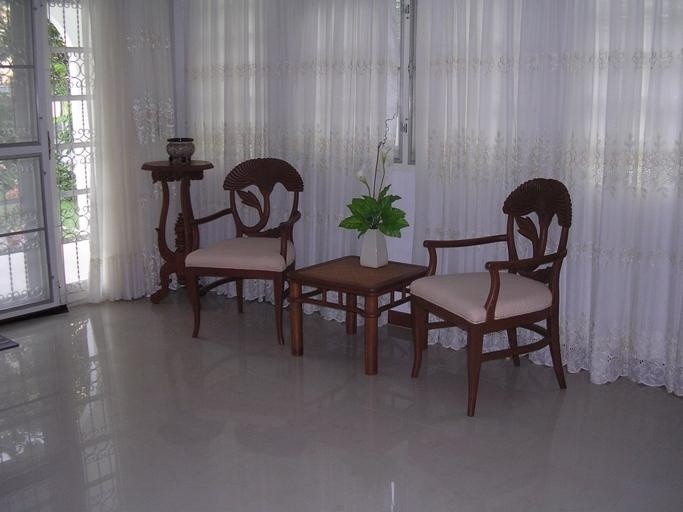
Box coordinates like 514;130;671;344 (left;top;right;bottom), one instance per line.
184;157;304;347
408;177;572;421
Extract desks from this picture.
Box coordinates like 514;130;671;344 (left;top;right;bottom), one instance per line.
292;254;428;377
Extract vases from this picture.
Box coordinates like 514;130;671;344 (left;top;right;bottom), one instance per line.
359;228;389;268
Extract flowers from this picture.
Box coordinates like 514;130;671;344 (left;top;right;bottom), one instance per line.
335;145;409;241
